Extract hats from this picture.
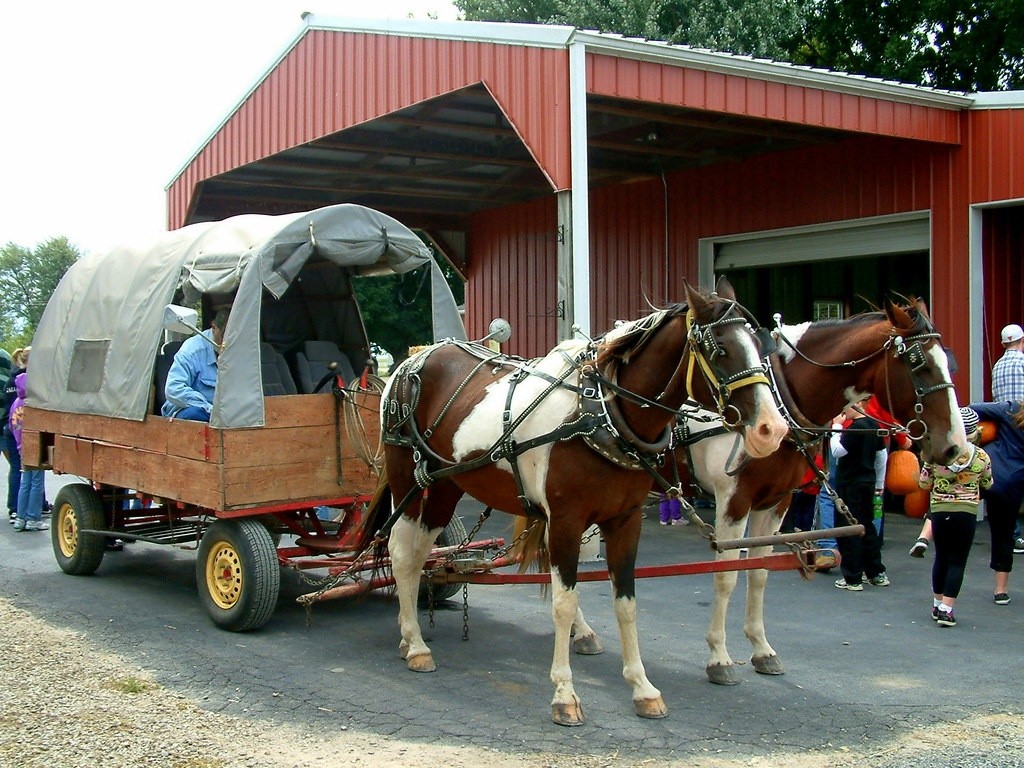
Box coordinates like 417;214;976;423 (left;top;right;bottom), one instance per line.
1002;325;1024;342
959;407;979;434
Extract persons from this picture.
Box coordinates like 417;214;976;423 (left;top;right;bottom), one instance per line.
161;307;230;422
0;345;55;532
991;323;1024;553
658;492;689;526
909;400;1024;605
918;406;994;626
739;388;913;591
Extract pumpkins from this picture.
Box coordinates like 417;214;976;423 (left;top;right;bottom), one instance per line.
977;420;997;444
885;449;930;518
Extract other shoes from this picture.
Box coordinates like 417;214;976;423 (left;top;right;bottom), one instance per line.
909;538;929;559
994;591;1011;604
816;555;838;566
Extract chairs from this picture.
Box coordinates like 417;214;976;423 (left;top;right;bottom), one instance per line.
294;341;356;394
260;342;297;396
153;341;183;417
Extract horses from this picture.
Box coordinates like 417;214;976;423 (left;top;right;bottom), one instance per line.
510;292;971;688
345;273;787;728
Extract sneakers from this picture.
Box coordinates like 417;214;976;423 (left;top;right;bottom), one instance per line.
660;521;667;525
835;578;863;590
41;504;54;518
938;609;956;625
931;605;939;619
25;520;49;529
9;513;18;523
862;572;890;585
14;518;26;529
672;517;689;526
1013;538;1024;552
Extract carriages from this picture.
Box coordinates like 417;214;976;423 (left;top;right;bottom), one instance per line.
19;198;968;728
